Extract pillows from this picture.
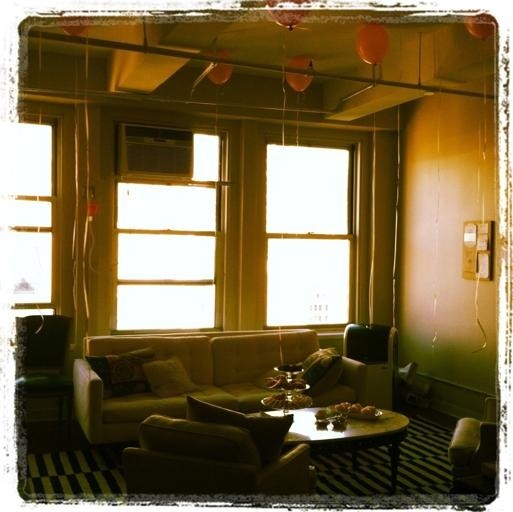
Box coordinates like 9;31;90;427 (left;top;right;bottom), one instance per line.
138;414;261;470
85;347;158;399
141;357;201;398
184;394;294;468
303;348;343;395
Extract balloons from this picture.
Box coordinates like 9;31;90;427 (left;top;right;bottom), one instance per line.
60;16;89;36
464;14;495;42
286;56;314;93
269;10;310;31
356;23;389;65
202;49;234;84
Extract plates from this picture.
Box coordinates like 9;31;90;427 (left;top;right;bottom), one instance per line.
328;404;384;420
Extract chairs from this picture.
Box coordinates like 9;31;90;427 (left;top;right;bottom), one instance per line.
121;413;317;495
16;313;73;447
448;397;497;495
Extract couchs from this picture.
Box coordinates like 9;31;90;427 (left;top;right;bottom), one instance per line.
74;329;367;443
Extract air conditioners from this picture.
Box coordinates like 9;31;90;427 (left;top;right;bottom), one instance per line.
118;123;193;181
